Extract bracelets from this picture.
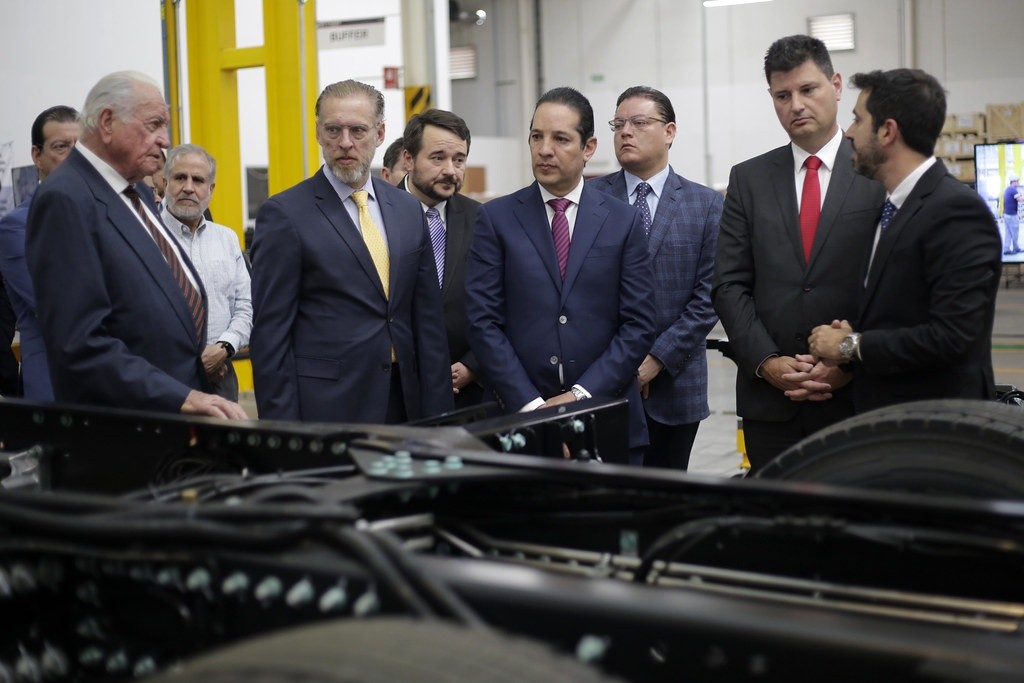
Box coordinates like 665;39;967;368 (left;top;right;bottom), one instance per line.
218;341;234;358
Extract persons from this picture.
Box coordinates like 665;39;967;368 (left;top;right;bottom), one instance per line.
459;87;657;467
809;70;1003;411
382;137;408;186
582;86;723;473
393;109;484;410
711;35;889;462
23;69;251;422
249;80;455;425
0;104;253;404
1002;175;1024;255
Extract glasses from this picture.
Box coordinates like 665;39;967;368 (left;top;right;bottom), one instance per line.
608;114;667;133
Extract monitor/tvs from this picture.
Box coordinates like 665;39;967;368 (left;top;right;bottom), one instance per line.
972;143;1024;264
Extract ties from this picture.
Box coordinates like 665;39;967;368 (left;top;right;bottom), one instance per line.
881;198;896;232
633;182;653;242
548;194;572;281
124;186;204;345
800;155;822;263
351;191;397;363
426;207;448;290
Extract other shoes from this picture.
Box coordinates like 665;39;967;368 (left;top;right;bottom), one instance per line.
1004;246;1024;255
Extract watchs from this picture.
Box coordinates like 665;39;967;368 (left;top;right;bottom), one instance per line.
839;332;860;359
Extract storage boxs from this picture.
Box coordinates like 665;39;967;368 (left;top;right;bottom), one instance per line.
933;133;986;159
985;102;1024;144
460;167;485;196
942;158;976;184
938;113;986;136
